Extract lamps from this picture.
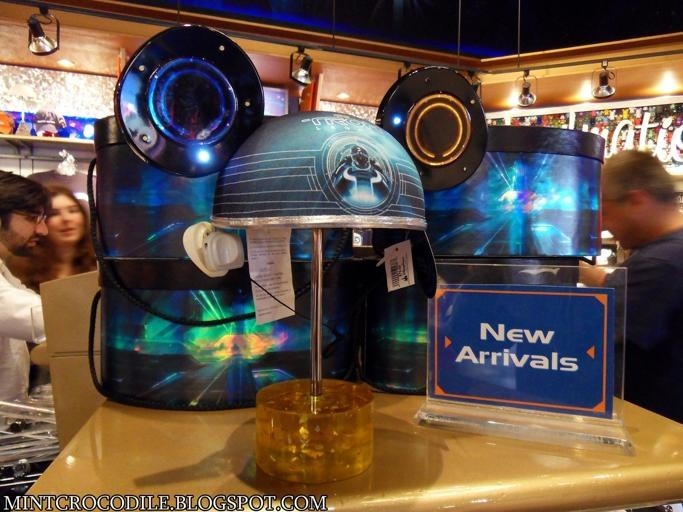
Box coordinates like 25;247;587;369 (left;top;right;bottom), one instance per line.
590;57;616;101
209;108;431;489
288;44;314;88
26;3;62;57
512;60;537;108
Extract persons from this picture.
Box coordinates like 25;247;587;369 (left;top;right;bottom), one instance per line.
575;149;683;425
3;182;97;295
0;167;51;430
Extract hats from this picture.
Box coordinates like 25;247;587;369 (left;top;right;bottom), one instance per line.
210;107;429;233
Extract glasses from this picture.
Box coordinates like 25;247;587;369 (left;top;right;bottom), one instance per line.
9;209;47;225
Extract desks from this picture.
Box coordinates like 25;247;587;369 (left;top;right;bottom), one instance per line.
17;393;682;512
